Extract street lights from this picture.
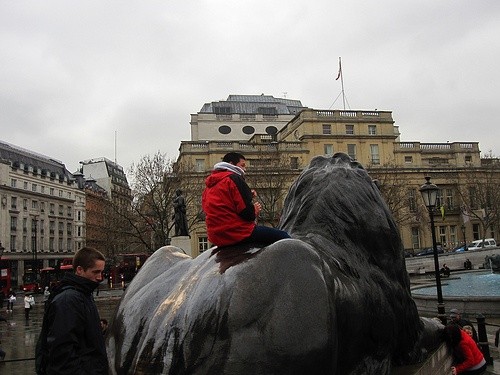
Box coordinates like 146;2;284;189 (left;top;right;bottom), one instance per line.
419;176;447;326
461;225;468;251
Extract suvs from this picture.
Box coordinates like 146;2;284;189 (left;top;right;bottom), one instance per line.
403;248;415;258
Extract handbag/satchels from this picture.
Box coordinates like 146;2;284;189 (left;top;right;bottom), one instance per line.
9;295;16;302
29;297;35;306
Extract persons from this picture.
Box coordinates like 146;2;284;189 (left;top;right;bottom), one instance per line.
99;319;108;335
35;247;114;375
202;152;291;249
439;264;450;278
7;288;16;312
24;293;35;320
43;286;51;305
170;189;189;237
443;325;487;375
464;259;472;270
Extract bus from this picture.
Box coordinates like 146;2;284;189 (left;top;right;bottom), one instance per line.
22;253;150;294
0;254;13;302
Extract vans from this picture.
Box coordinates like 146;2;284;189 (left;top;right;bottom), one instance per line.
467;238;497;252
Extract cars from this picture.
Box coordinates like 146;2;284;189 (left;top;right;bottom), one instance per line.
452;243;470;253
416;245;448;257
